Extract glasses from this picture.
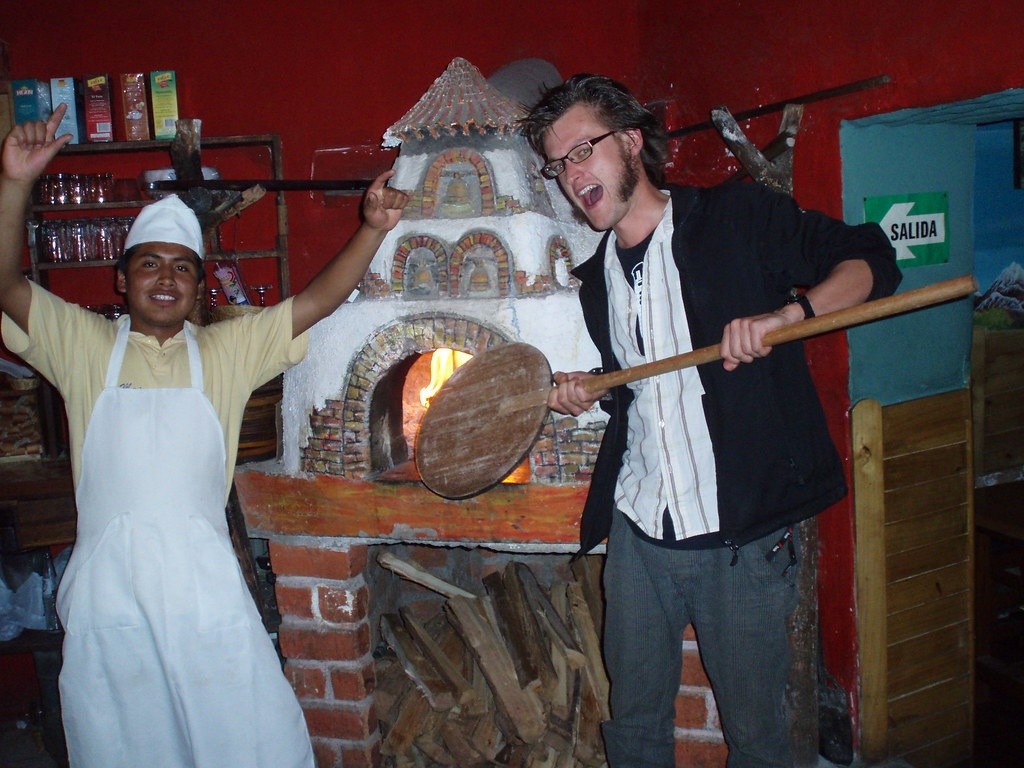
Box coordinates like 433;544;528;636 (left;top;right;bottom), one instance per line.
542;130;618;180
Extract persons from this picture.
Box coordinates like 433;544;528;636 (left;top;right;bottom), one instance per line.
0;102;413;768
516;72;903;768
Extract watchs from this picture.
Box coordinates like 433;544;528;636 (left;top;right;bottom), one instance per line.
784;294;815;320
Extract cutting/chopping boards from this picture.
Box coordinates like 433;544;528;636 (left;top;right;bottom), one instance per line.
412;274;975;499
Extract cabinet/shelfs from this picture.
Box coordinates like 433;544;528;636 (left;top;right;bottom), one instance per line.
22;134;291;304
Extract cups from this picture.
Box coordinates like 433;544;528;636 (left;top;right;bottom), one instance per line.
38;216;138;262
32;173;116;204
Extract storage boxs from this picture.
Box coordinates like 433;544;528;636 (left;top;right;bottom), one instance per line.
10;70;180;144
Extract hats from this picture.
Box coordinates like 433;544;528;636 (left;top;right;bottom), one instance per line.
123;194;204;261
